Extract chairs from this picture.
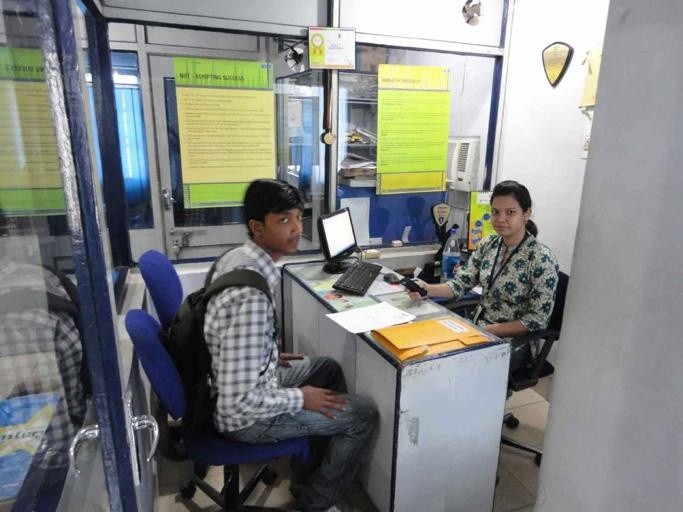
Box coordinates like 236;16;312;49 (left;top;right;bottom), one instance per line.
436;270;569;487
124;309;306;512
138;249;184;331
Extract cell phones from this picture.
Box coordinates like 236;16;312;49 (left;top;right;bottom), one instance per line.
400;277;427;297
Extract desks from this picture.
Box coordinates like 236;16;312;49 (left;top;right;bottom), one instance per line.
281;260;512;512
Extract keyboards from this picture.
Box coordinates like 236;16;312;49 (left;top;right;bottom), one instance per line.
332;261;382;296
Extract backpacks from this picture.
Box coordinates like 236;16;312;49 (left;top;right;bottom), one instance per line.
165;243;271;426
1;265;91;399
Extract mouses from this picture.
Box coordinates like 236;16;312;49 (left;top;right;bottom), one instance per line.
384;273;400;284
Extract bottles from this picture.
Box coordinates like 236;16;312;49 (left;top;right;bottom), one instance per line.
441;229;461;282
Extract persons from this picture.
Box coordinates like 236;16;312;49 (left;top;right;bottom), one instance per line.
408;181;559;377
197;180;378;511
1;208;87;511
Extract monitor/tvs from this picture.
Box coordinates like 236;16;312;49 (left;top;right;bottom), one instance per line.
317;207;358;274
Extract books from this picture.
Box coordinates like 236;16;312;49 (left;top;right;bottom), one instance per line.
324;302;415;335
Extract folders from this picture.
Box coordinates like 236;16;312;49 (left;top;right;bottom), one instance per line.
369;316;494;362
